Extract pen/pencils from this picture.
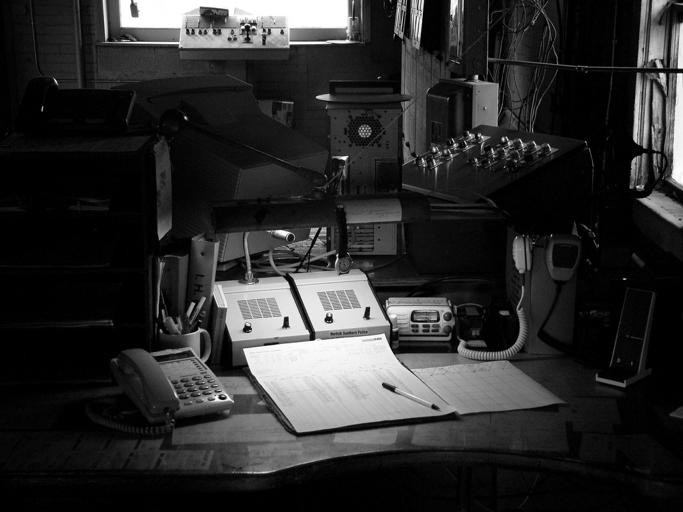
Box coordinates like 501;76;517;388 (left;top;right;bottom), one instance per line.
382;383;440;410
153;296;206;335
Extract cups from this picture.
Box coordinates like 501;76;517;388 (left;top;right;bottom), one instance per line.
161;325;212;364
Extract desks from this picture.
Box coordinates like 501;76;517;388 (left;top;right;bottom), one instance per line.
0;354;683;512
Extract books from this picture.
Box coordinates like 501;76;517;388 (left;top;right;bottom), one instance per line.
239;329;455;438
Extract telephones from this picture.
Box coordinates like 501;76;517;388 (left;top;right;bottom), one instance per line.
110;347;235;423
